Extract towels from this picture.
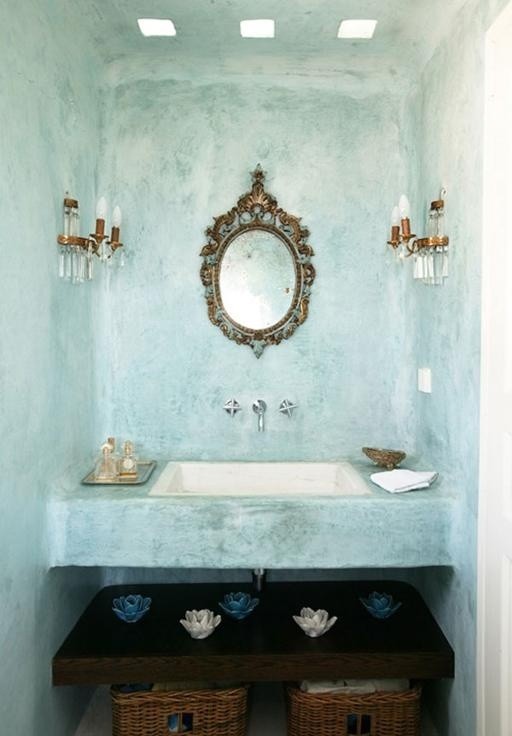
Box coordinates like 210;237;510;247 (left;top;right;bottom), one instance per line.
413;470;440;486
368;468;430;495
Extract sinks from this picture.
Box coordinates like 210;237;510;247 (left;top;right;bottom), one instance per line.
147;458;373;500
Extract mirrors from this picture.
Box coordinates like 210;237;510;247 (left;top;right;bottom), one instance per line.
198;160;318;359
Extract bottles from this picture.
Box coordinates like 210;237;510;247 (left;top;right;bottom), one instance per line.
58;245;93;284
420;245;448;285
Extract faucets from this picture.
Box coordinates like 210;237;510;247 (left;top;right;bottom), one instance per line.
252;400;267;434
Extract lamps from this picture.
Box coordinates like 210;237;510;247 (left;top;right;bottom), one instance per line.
57;188;122;285
385;187;451;288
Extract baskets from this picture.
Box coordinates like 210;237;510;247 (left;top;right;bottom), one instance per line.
109;684;248;736
285;684;422;736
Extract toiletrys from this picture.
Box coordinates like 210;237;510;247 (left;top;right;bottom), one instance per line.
94;444;118;480
96;437;139;483
118;441;138;478
